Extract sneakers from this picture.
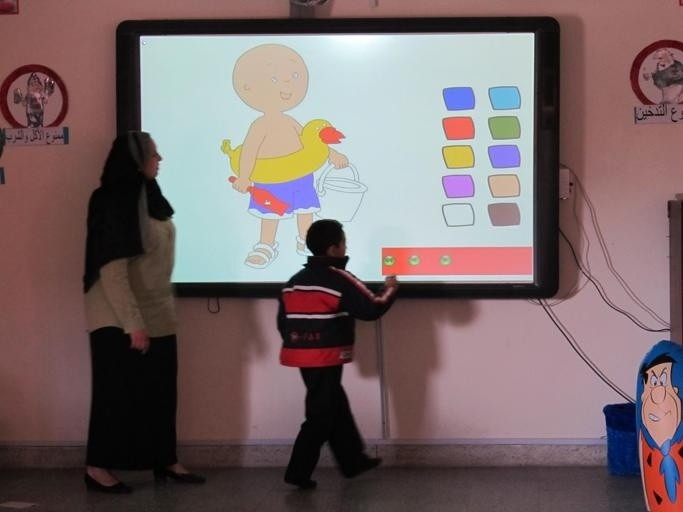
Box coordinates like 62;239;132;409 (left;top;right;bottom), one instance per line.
340;457;382;478
284;474;318;489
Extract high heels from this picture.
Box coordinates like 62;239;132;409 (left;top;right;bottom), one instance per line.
154;465;206;484
84;471;133;494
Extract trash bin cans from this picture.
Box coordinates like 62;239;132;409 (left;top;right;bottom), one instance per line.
603;402;640;476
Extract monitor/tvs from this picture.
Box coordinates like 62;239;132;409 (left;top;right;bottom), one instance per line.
112;15;562;300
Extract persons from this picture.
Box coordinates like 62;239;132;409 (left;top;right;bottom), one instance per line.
273;218;400;490
79;129;208;495
